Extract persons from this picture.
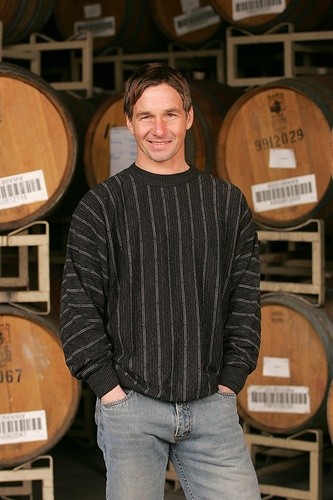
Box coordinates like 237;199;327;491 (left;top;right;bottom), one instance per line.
58;63;262;500
